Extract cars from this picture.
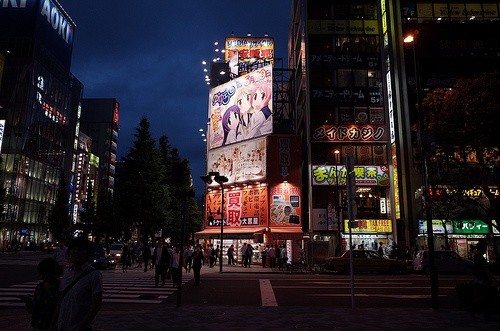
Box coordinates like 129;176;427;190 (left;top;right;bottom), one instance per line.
43;243;59;253
89;245;117;271
109;243;127;263
323;250;404;276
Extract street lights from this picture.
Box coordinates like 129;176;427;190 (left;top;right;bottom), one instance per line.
333;149;341;253
175;188;196;309
403;34;442;311
199;171;228;272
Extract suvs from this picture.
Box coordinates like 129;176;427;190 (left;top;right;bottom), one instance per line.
413;250;475;276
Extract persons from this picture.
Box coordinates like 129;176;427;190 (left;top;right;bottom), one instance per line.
440;245;447;250
120;240;220;290
21;241;103;331
241;243;252;267
389;242;411;261
228;244;235;265
412;245;425;258
378;242;383;254
268;243;287;268
